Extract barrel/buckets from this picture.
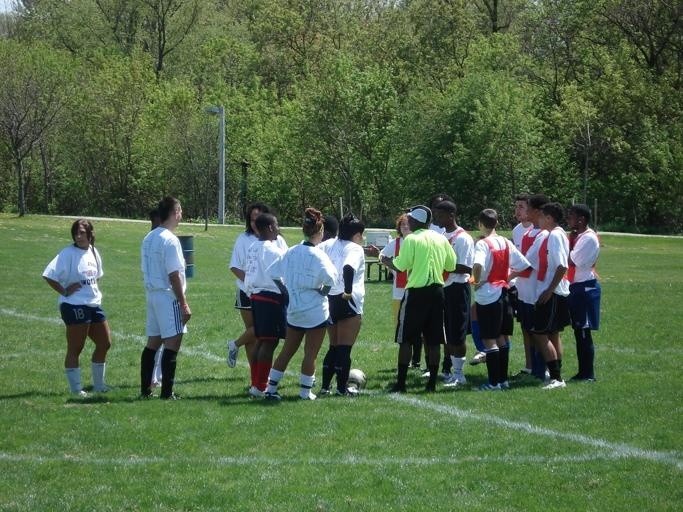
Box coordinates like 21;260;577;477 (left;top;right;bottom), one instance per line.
176;234;194;279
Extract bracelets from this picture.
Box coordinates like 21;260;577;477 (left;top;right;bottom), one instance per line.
63;289;67;298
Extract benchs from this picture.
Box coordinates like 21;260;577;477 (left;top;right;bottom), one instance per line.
362;246;390;282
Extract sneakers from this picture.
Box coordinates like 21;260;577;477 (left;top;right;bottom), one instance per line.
335;390;353;397
411;361;465;392
570;374;595;382
320;389;330;396
471;380;508;392
93;385;111;392
69;391;86;396
516;368;565;391
297;393;316;401
469;351;486;364
227;340;238;368
248;377;280;400
389;384;404;393
139;381;181;400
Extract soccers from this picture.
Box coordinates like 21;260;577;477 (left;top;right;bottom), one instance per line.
346;368;367;390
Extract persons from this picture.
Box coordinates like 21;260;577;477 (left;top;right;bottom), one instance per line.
42;219;112;401
228;202;366;400
149;207;164;386
363;198;598;393
140;196;192;400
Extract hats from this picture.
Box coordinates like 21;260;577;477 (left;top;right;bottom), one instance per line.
405;205;432;224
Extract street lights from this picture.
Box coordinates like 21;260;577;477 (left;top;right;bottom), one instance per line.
206;103;226;225
